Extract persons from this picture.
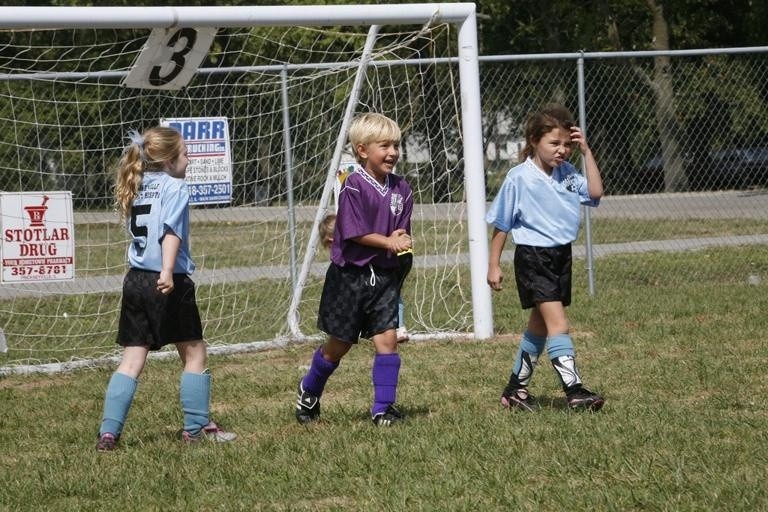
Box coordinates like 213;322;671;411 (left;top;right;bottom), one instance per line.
292;108;414;427
95;124;240;450
483;102;606;413
318;215;410;342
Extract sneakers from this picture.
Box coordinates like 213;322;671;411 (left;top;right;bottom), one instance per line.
295;377;321;426
396;326;409;343
181;420;237;444
370;408;395;427
501;386;541;415
567;388;604;412
96;431;121;453
